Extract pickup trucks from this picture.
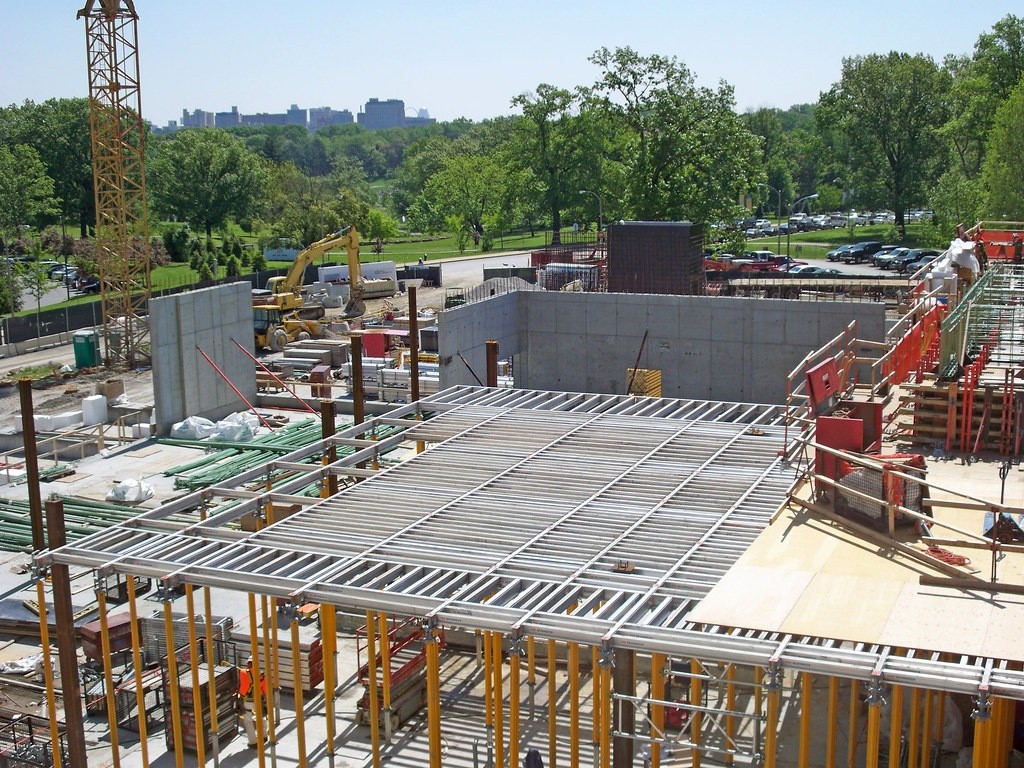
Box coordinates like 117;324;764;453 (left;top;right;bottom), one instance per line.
839;242;900;265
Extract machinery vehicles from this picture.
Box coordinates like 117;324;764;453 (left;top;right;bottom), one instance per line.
252;222;367;320
253;305;322;351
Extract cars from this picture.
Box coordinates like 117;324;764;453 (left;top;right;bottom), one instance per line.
877;247;909;268
712;210;933;242
825;245;854;262
872;251;891;267
3;257;127;294
906;255;939;274
703;246;842;275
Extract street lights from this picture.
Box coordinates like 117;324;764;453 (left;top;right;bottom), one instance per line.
579;190;602;231
785;192;819;276
757;182;781;256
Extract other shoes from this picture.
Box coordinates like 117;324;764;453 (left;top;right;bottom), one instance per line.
247;743;258;749
264;737;267;743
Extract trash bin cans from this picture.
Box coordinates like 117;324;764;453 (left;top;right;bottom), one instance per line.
73;331;102;369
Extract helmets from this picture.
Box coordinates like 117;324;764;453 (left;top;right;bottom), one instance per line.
246;655;253;663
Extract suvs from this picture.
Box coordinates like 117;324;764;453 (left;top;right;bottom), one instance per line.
891;249;941;271
